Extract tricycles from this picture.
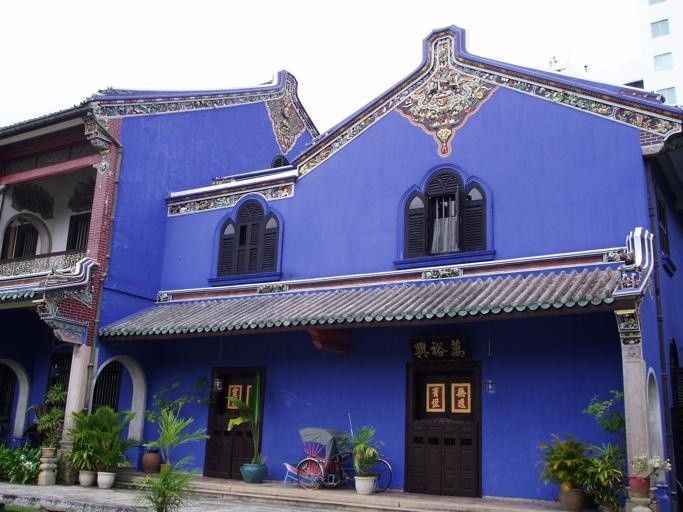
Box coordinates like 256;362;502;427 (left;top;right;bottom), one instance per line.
297;426;392;494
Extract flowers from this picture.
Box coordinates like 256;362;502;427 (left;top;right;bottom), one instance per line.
630;449;672;479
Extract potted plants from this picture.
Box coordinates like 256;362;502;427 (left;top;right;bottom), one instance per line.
0;374;217;489
225;372;270;483
532;430;629;512
329;421;386;495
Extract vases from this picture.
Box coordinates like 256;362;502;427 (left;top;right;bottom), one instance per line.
628;476;652;499
